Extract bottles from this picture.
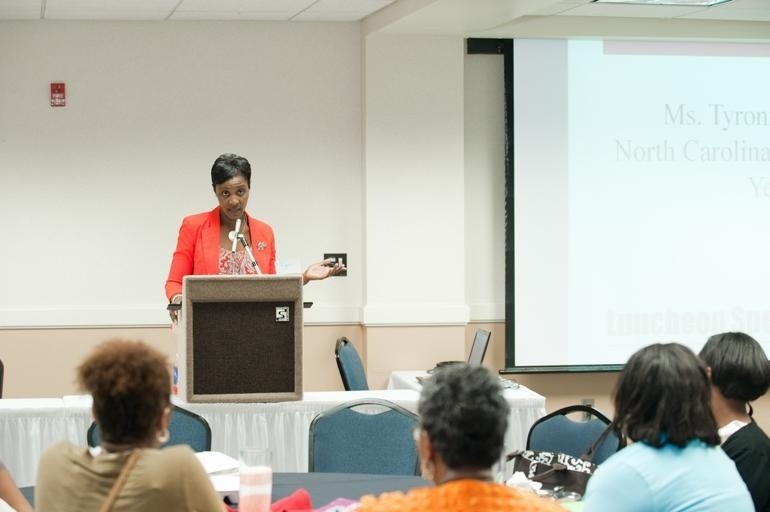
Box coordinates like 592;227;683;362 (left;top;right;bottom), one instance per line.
172;353;178;395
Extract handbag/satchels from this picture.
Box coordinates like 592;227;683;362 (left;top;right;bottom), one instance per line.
513;450;599;497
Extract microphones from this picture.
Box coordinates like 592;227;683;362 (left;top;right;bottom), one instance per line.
231;218;241;254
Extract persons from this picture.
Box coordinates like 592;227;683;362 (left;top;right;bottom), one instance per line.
352;362;575;512
162;153;349;326
0;361;33;512
695;332;770;512
578;341;755;511
32;336;227;511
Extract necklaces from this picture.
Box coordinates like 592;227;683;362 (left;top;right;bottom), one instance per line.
238;215;247;242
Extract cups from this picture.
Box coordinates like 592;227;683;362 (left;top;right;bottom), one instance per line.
236;447;275;512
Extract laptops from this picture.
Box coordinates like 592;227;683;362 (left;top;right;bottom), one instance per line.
415;327;492;384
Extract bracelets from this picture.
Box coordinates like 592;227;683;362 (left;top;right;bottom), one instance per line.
171;294;183;305
